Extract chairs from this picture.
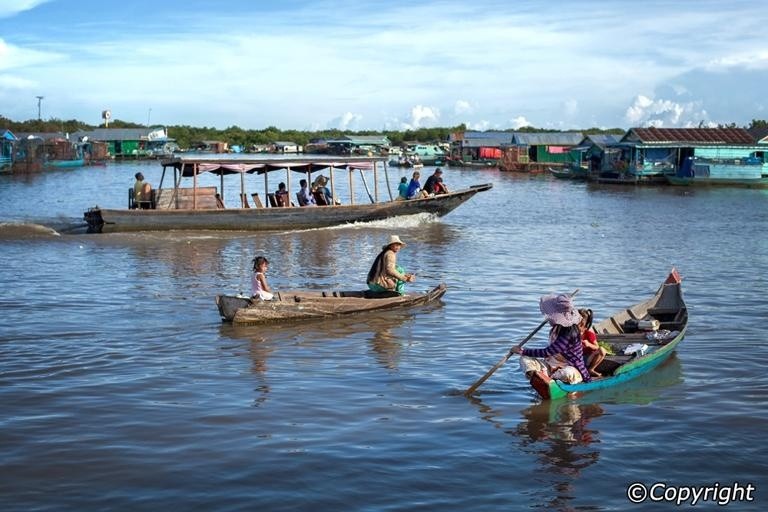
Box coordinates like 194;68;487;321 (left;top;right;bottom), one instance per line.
296;192;306;205
312;191;327;205
217;194;226;208
252;193;263;208
268;192;277;207
129;188;152;209
240;193;251;208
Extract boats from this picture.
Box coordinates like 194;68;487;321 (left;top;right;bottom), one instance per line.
82;184;498;235
213;283;448;324
515;268;691;403
217;300;444;340
535;348;684;426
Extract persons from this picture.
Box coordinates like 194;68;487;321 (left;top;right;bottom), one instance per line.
365;234;415;297
406;171;421;199
310;181;318;193
394;176;409;201
250;255;275;301
423;167;449;195
435;177;447;193
275;182;294;207
299;178;318;207
315;173;341;206
134;171;151;210
573;308;607;378
509;293;592;385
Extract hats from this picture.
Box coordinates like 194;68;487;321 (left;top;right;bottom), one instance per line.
381;234;406;250
539;292;584;328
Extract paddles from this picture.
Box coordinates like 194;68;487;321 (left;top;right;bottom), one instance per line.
463;289;581;398
